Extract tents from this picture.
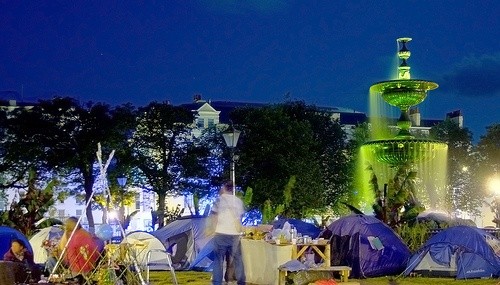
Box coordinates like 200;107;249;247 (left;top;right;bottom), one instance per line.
408;225;500;279
28;224;64;264
309;214;412;279
119;214;217;272
272;219;320;239
0;226;34;262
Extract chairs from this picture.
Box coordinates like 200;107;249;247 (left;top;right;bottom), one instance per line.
146;249;178;285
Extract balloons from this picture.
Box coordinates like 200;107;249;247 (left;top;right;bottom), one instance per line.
92;236;104;252
97;224;113;241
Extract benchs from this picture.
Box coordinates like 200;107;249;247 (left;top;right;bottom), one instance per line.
278;265;352;285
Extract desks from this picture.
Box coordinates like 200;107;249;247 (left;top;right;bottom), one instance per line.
222;238;331;285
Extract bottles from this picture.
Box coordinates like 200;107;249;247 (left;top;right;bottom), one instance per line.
290;224;295;242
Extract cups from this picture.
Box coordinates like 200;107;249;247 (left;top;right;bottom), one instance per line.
292;235;324;244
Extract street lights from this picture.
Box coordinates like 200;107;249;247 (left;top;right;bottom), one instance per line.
117;173;128;243
222;123;242;195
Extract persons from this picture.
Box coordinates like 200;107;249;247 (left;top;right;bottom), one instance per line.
3;238;41;285
43;216;97;281
210;178;247;285
